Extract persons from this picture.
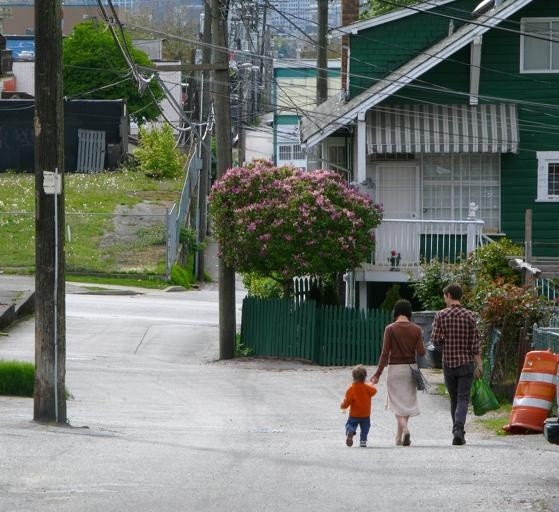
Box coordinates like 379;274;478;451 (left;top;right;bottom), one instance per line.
340;283;483;448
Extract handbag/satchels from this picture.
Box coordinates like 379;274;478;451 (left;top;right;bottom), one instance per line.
470;369;500;416
411;368;431;393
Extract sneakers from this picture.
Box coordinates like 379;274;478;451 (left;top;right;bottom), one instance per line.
346;431;353;447
396;431;411;446
452;430;466;445
360;440;366;447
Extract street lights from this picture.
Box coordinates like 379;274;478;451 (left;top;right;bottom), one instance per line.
238;58;252;164
